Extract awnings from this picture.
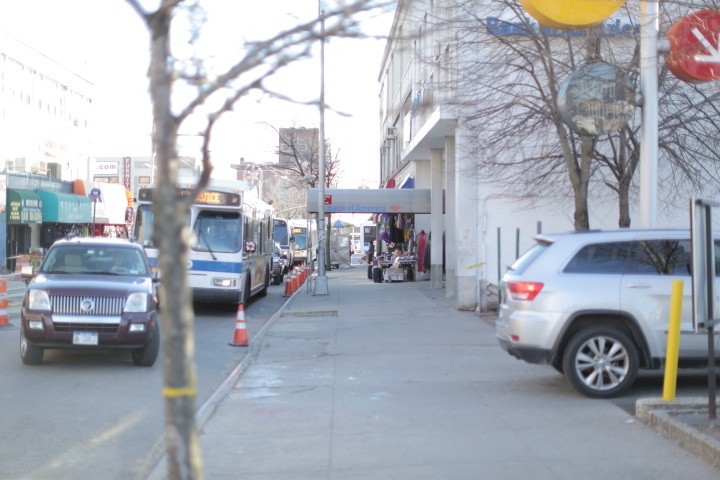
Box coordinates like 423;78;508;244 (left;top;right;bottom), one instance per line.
6;188;42;224
37;188;91;223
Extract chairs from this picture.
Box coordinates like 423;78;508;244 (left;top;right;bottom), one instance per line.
590;245;691;274
56;254;88;272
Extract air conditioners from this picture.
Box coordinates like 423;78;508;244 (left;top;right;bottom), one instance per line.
387;127;397;140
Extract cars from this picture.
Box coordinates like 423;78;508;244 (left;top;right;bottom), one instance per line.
269;241;288;286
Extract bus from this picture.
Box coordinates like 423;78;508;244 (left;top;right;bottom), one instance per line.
291;224;318;265
132;173;274;311
273;217;297;273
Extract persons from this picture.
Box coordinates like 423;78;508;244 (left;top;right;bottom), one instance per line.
367;240;374;264
211;221;235;249
108;251;132;272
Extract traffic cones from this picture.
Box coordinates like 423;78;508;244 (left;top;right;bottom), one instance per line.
227;304;249;347
282;258;315;298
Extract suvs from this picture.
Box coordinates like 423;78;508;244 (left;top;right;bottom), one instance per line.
494;228;720;399
19;234;161;367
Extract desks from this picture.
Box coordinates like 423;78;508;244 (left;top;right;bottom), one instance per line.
381;260;415;282
